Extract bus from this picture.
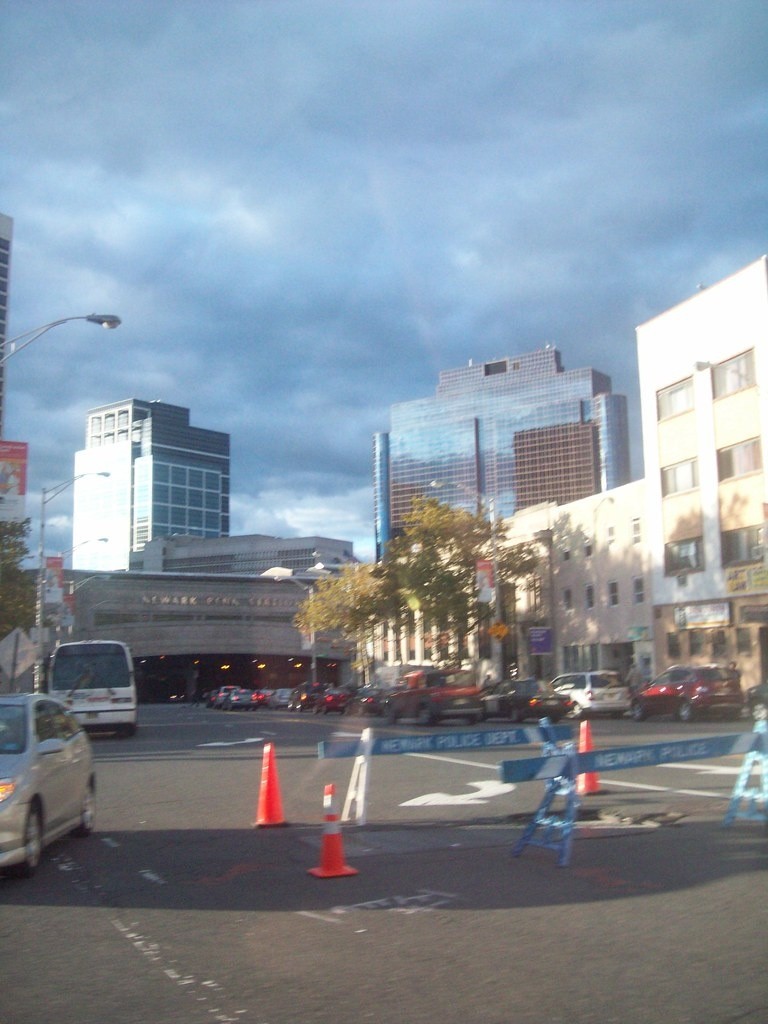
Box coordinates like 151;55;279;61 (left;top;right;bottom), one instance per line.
47;638;138;738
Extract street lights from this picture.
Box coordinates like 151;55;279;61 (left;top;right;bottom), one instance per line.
273;575;317;681
34;469;114;695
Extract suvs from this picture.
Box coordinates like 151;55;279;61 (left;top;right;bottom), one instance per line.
630;663;743;723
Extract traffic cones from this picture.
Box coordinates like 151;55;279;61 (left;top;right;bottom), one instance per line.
252;741;292;828
306;784;360;878
575;719;607;796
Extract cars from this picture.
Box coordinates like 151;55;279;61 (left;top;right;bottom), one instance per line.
748;681;768;721
482;677;573;723
0;693;96;877
548;670;631;719
385;670;482;728
208;681;398;717
